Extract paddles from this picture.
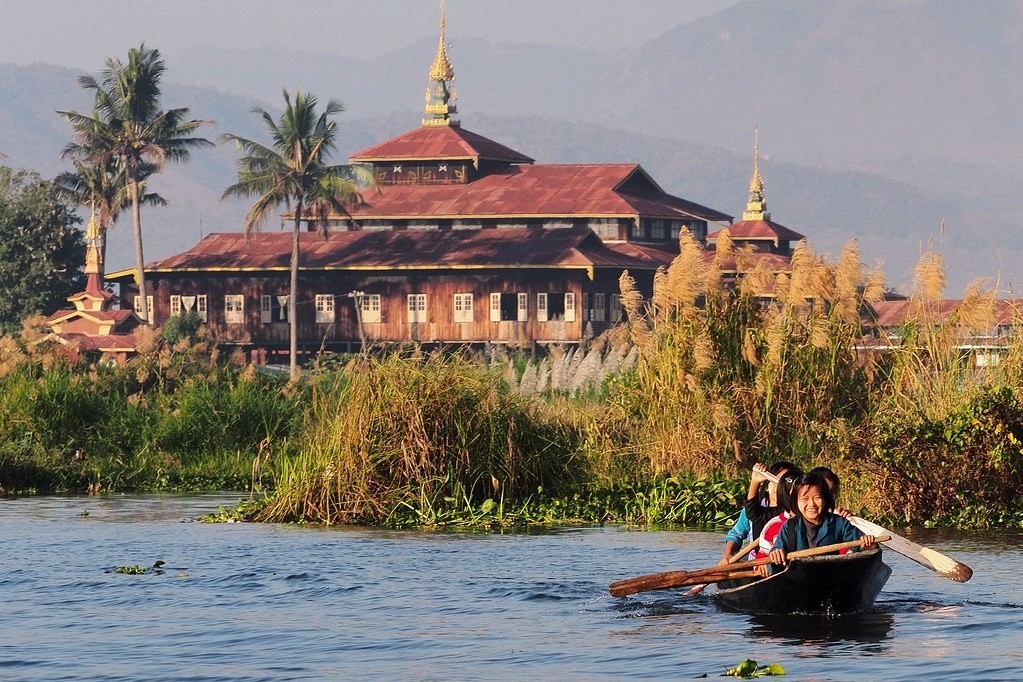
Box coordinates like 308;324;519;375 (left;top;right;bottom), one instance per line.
686;537;760;597
679;569;761;587
752;464;974;584
609;535;892;597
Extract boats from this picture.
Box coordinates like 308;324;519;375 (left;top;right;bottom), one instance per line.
709;546;893;620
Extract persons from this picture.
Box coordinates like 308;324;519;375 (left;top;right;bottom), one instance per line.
720;461;878;590
